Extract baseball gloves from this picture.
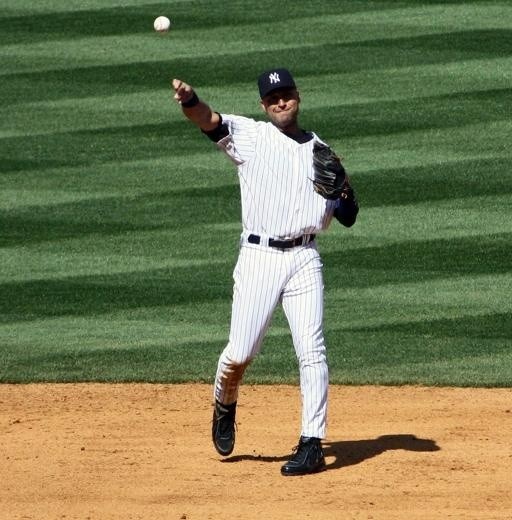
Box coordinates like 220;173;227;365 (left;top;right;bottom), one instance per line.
307;141;348;200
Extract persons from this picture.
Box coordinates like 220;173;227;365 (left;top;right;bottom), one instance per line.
173;68;358;477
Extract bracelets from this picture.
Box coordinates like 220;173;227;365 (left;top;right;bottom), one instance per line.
182;92;199;108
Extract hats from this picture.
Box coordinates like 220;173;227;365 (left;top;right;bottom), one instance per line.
257;68;297;99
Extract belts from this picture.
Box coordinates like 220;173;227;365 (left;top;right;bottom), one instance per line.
247;234;316;249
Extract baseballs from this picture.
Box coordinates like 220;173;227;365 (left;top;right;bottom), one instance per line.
153;16;170;32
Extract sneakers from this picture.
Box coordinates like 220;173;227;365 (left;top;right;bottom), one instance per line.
281;437;327;476
213;399;237;456
